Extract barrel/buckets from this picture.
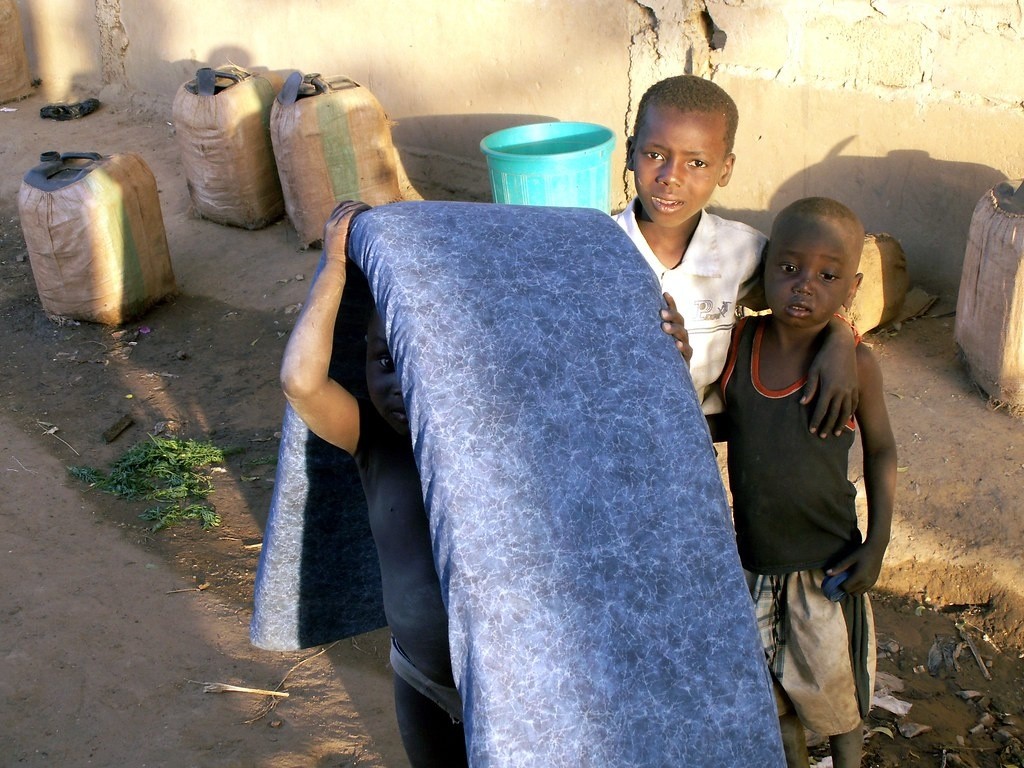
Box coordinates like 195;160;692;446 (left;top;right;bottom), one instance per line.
479;121;618;218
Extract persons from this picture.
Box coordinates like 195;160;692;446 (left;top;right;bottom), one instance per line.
278;203;468;768
659;196;897;768
599;77;861;441
